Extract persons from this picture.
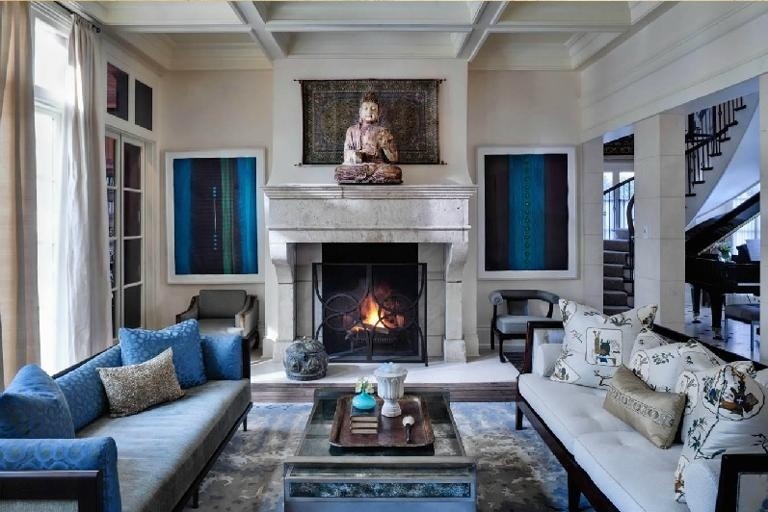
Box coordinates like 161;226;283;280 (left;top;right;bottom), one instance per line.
334;91;401;185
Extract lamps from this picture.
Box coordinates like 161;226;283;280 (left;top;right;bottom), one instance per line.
486;289;562;363
174;289;260;353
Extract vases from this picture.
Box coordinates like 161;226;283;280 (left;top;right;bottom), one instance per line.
353;375;375;395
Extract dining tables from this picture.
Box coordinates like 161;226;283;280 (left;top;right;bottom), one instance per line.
716;244;733;264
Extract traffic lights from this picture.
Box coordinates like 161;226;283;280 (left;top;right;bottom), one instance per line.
92;344;188;420
0;362;76;442
628;322;732;401
548;291;662;394
600;360;689;451
116;316;210;390
675;358;756;446
672;362;768;507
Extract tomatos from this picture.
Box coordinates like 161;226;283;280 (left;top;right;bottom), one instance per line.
684;192;759;341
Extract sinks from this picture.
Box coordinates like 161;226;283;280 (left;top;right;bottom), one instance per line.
0;333;255;511
512;312;768;511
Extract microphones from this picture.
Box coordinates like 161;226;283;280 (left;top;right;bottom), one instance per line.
721;303;761;353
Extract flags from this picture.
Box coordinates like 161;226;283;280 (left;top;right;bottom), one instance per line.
351;387;378;411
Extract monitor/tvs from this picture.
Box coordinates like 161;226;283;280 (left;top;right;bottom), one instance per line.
349;404;380;435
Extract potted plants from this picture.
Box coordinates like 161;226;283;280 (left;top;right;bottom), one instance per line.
162;143;274;287
470;142;581;284
